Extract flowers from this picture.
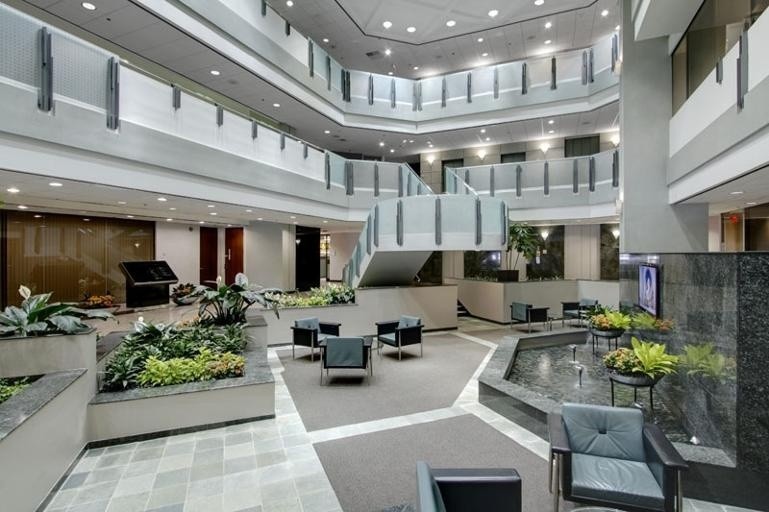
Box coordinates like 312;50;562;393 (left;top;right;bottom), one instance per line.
603;335;680;381
588;307;633;331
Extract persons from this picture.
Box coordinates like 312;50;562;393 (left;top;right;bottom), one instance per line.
643;268;654;311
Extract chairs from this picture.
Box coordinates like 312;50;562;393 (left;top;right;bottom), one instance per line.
509;298;598;334
415;400;689;511
289;315;425;387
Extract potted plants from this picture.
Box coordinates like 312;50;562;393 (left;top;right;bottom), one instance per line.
493;222;545;281
174;274;269;349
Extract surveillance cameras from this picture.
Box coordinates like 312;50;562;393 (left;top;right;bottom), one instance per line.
189;227;193;231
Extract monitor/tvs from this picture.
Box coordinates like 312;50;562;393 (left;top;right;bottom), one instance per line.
637;262;660;321
480;251;501;267
